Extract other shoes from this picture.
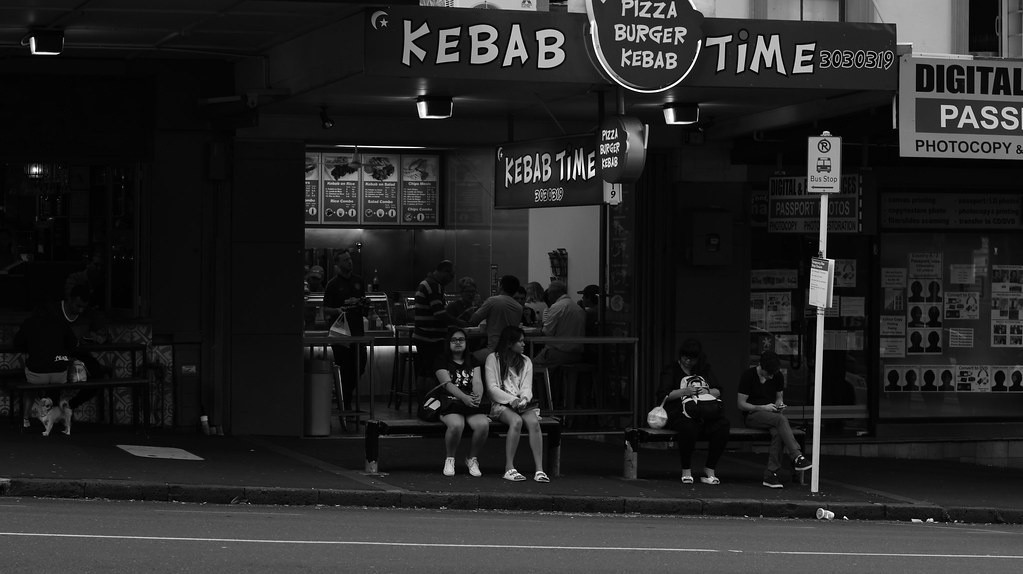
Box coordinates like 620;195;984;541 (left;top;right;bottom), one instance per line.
763;476;783;488
794;456;812;470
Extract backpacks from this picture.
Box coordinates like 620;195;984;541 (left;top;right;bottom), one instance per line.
680;374;722;418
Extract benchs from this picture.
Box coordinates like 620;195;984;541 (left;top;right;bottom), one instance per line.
786;401;869;418
364;417;562;479
6;376;150;440
623;428;807;485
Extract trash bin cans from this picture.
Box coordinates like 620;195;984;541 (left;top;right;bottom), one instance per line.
305;358;334;435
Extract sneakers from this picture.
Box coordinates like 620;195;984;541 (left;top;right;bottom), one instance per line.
465;456;482;476
443;457;455;476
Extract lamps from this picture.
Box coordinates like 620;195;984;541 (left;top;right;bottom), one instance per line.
25;31;65;55
662;102;699;125
416;96;454;119
319;104;333;126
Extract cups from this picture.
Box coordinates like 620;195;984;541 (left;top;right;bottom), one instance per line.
815;508;835;520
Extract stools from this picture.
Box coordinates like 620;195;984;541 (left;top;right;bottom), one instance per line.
552;362;608;430
395;352;417;411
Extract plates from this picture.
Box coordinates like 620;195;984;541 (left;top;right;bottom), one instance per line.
305;152;439;185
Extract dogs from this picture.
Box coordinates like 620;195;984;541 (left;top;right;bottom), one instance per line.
37;397;72;435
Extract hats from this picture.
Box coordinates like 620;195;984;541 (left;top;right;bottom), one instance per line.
577;285;599;296
309;266;324;279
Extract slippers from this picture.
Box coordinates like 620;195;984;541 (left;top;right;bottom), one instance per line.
533;471;550;483
681;476;694;484
501;469;526;481
701;476;720;485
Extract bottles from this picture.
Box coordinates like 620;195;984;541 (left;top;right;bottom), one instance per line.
368;306;383;330
372;271;380;292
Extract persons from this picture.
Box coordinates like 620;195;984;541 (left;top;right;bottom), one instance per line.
485;326;551;483
432;328;488;476
323;248;384;419
414;260;467;420
13;284;103;431
524;282;548;321
532;281;586;388
449;276;480;321
657;340;730;484
512;287;535;326
736;350;811;487
471;276;523;364
577;285;609;364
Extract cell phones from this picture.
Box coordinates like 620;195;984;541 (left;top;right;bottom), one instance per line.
776;406;786;410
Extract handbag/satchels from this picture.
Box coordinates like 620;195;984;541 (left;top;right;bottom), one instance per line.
647;395;669;429
417;381;456;422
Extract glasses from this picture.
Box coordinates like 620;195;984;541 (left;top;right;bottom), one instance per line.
448;337;465;344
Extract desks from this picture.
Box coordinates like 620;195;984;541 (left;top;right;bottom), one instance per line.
0;342;147;380
304;325;638;430
